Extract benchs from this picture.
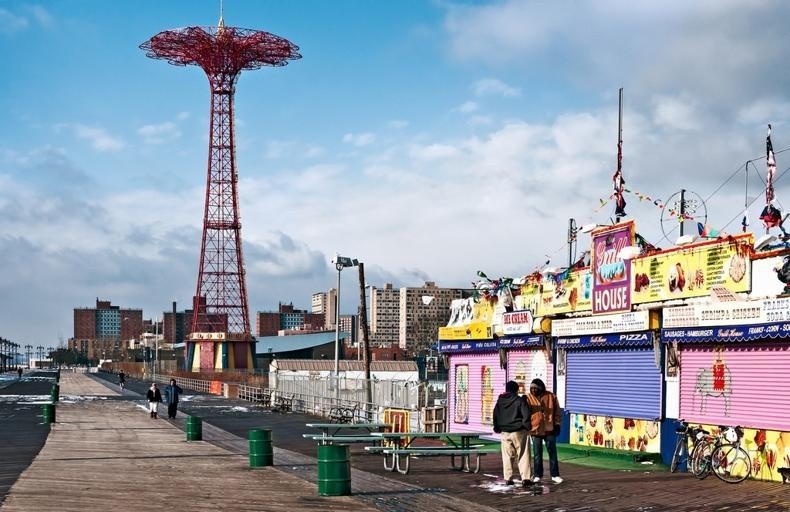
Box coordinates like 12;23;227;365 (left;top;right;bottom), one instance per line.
330;403;358;423
301;422;501;475
253;390;272;407
273;393;296;414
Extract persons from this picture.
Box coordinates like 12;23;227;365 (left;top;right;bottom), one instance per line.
118;370;126;390
147;383;162;420
17;367;23;379
164;379;183;419
675;263;686;291
522;379;564;484
493;380;537;487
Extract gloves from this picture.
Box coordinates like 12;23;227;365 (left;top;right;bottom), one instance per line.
554;424;561;437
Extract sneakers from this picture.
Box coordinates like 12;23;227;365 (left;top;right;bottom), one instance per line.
551;475;564;484
533;476;541;483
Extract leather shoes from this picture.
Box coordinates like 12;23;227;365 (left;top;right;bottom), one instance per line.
521;479;535;486
505;480;514;485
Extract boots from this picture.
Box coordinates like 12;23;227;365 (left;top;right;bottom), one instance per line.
150;411;157;419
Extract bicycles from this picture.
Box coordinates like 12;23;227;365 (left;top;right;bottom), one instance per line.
671;417;751;484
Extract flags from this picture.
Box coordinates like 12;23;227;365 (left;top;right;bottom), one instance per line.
765;124;777;203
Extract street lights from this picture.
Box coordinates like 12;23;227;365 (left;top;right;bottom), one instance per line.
0;337;81;376
153;315;162;362
329;254;354;414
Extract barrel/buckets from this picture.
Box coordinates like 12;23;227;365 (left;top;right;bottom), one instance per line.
187;416;202;441
249;428;273;468
43;405;55;425
317;444;351;496
51;385;60;401
